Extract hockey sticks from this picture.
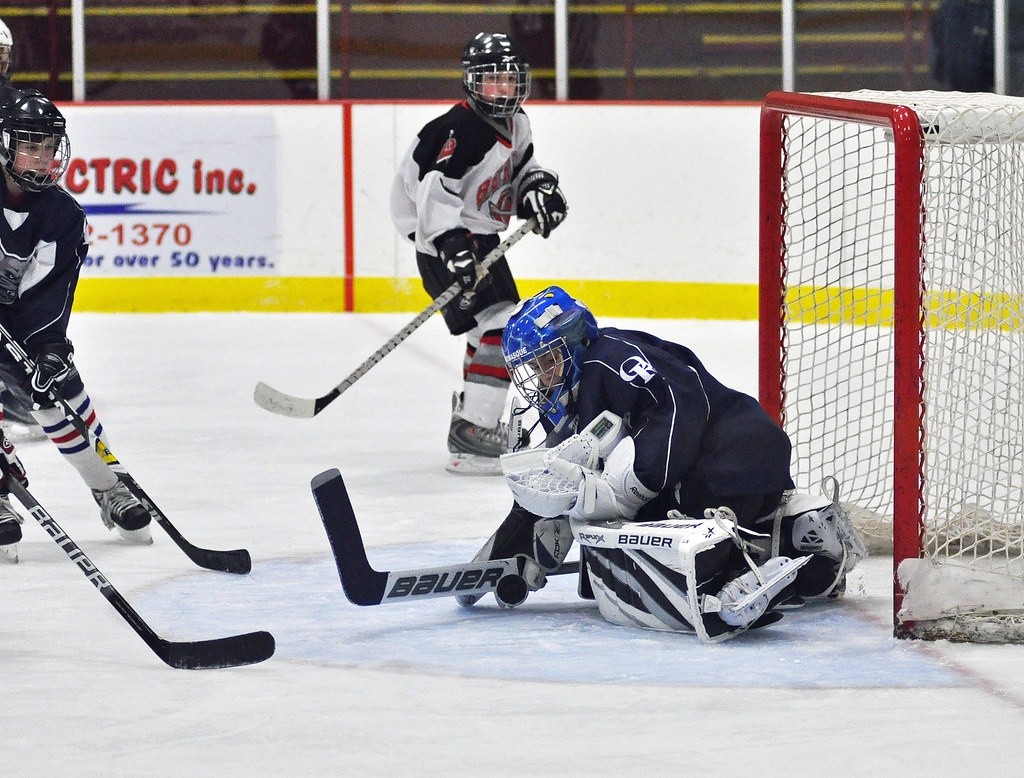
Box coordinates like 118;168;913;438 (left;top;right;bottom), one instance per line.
1;460;276;673
0;316;254;577
252;216;536;420
310;465;579;609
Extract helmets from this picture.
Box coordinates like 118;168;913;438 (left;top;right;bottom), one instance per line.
501;285;600;434
0;19;17;84
460;29;531;120
0;87;71;192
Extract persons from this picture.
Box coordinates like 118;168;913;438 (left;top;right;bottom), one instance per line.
0;20;13;82
0;89;154;562
390;28;568;476
467;284;848;641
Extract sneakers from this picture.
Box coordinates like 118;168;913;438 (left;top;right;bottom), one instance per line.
91;476;153;545
0;486;24;563
445;391;531;475
0;379;48;444
792;501;869;573
716;553;815;628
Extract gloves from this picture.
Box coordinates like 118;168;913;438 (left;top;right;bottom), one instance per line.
17;337;74;410
517;168;569;239
432;228;495;310
